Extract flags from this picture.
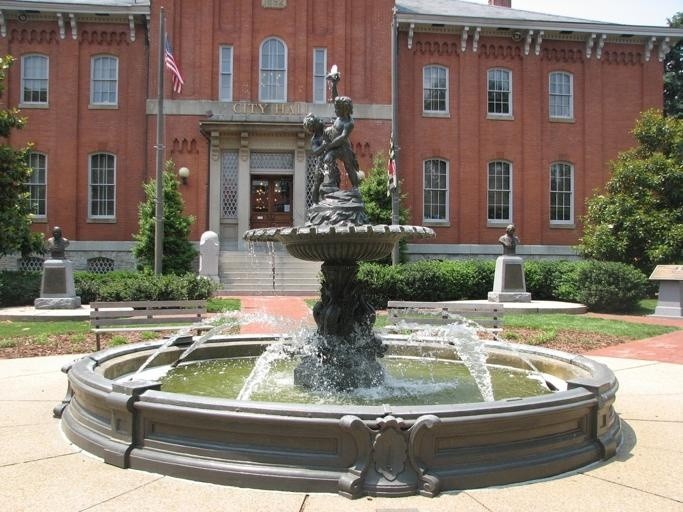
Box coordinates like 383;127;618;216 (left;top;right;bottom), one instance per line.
164;30;184;95
386;133;397;199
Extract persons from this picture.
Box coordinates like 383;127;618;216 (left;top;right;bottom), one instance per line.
303;112;340;204
498;225;519;248
322;73;361;187
48;226;70;252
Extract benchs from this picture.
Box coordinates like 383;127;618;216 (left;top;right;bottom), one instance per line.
90;299;213;354
387;301;503;344
